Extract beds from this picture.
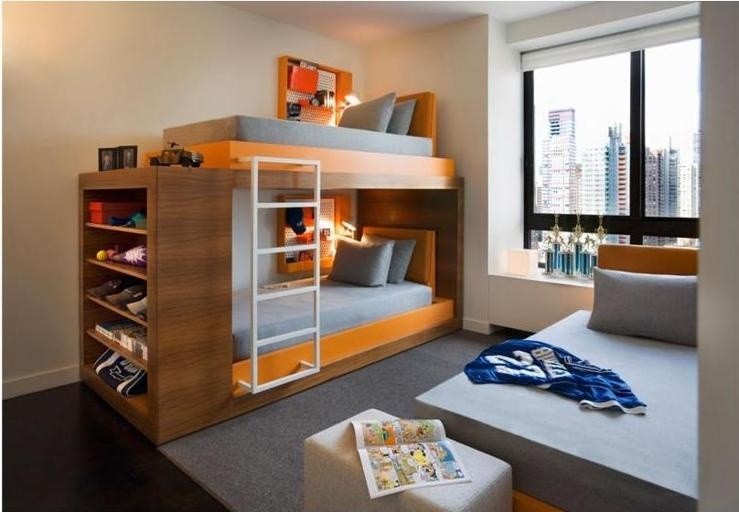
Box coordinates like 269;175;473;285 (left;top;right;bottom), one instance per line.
144;91;465;417
415;244;698;512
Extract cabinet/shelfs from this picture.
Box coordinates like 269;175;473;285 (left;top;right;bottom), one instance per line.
278;56;352;126
78;166;233;446
277;195;351;273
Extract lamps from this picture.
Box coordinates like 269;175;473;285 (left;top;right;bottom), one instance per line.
538;213;607;282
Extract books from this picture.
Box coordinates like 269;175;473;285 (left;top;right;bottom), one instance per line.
298;58;320;71
316;90;335;109
348;416;474;501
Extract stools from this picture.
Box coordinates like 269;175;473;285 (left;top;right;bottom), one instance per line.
302;405;513;511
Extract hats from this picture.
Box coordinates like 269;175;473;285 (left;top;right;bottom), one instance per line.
86;281;148;317
286;208;306;233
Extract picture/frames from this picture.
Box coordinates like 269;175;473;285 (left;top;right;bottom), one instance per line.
118;146;137;168
99;148;116;170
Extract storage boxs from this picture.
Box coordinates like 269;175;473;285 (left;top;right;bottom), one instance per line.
89;201;144;224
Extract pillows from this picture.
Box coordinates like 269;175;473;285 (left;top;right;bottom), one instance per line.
338;91;397;132
586;267;697;347
386;98;417;135
361;233;416;284
327;234;397;287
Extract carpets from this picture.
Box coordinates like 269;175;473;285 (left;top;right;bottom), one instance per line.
155;327;506;511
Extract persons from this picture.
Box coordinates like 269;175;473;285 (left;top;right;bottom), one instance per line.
102;152;113;171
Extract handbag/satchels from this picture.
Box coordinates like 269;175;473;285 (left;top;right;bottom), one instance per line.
92;349;147;396
